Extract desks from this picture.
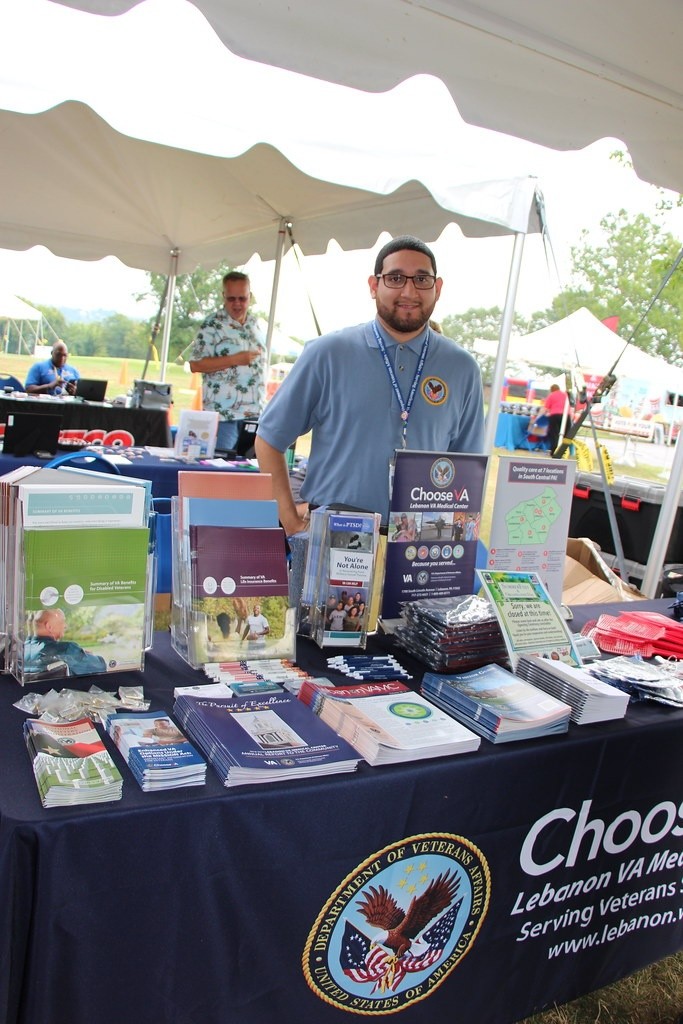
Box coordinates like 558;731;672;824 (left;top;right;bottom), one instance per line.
0;443;260;498
495;413;552;452
580;424;648;466
0;596;683;1024
0;388;173;449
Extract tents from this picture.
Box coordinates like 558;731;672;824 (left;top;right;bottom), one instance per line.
49;0;683;596
473;308;683;458
0;0;547;465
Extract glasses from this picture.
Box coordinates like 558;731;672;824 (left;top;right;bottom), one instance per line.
376;273;437;290
224;293;250;302
52;352;71;357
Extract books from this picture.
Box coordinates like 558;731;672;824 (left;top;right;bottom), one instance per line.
0;465;296;686
22;679;365;809
297;657;631;766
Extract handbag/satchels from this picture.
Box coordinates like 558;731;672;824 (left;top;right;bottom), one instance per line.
134;379;173;410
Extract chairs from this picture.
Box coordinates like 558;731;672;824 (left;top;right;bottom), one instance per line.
42;452;121;476
228;421;260;460
0;373;25;393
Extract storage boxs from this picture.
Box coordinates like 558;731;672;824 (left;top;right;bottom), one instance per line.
561;538;650;607
566;471;683;567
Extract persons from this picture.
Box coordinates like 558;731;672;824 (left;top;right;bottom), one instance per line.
114;725;154;746
144;720;189;747
539;384;572;456
254;236;485;545
348;535;361;549
435;515;446;536
393;512;419;542
321;591;365;632
189;273;267;450
453;513;480;540
31;610;107;673
239;605;270;652
25;613;34;673
25;342;79;397
